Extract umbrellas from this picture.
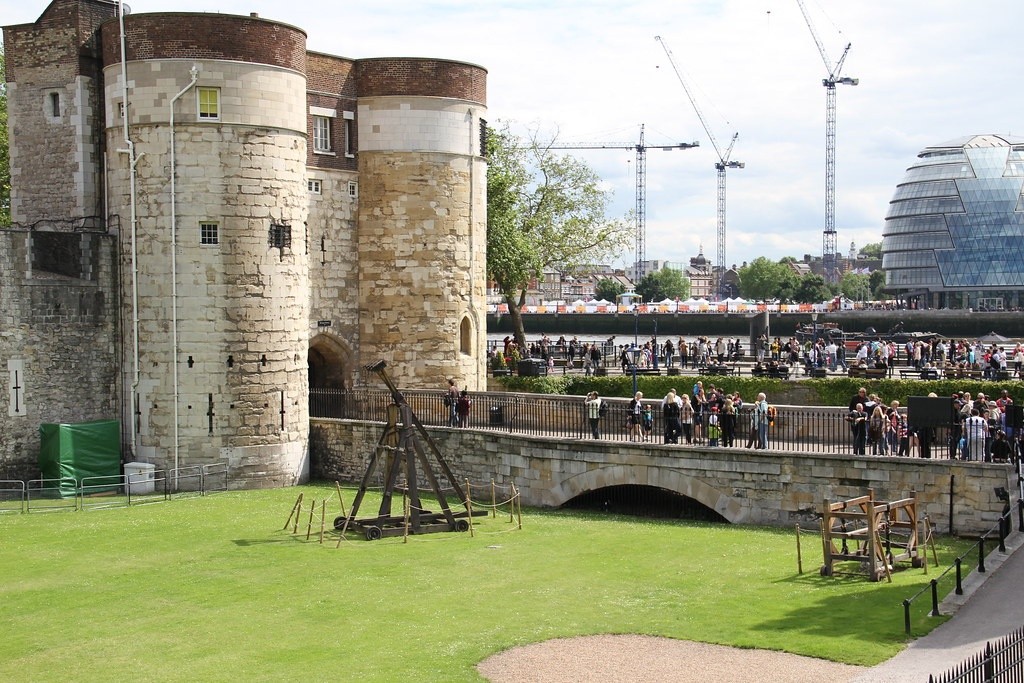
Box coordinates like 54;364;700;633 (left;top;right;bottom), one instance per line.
573;299;614;307
660;297;748;303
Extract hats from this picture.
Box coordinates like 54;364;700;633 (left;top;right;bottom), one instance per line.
711;407;720;412
988;400;998;407
954;399;964;406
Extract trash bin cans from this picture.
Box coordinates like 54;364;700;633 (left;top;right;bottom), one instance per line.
517;357;548;377
489;402;511;429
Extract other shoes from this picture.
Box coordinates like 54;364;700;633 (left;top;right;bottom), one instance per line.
670;437;677;444
693;439;704;444
631;438;637;442
641;435;650;441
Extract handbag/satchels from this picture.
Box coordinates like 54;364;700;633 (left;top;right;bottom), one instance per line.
626;419;633;429
455;402;460;412
567;362;574;369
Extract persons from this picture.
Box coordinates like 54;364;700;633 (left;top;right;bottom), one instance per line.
627;391;648;441
679;393;695;444
844;387;1023;465
706;384;768;449
457;391;471;428
584;391;602;439
662;388;683;444
443;379;459;427
904;336;1023;382
692;381;710;405
663;392;681;444
642;405;654;442
690;389;708;444
491;332;900;380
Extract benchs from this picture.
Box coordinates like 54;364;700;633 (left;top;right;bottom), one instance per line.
848;367;887;379
943;368;982;381
698;369;737;376
547;366;568;375
751;369;790;380
626;368;661;376
899;370;939;380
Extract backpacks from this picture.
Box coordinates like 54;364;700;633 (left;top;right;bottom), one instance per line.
599;401;608;417
759;403;777;427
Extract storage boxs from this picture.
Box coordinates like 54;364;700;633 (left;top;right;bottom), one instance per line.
124;462;155;494
38;417;121;500
